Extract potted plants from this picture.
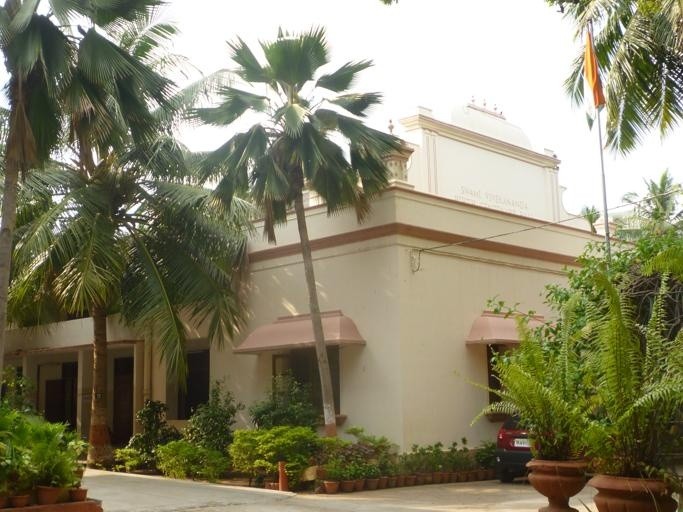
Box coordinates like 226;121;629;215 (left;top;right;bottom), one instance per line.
537;261;682;512
6;441;32;510
110;421;330;493
25;436;74;503
467;294;594;511
0;451;9;511
326;437;500;494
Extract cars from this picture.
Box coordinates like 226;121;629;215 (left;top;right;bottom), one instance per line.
494;407;614;480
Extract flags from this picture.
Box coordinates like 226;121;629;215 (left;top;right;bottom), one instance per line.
582;21;608;111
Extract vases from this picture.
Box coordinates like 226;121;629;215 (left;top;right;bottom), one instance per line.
70;491;89;502
